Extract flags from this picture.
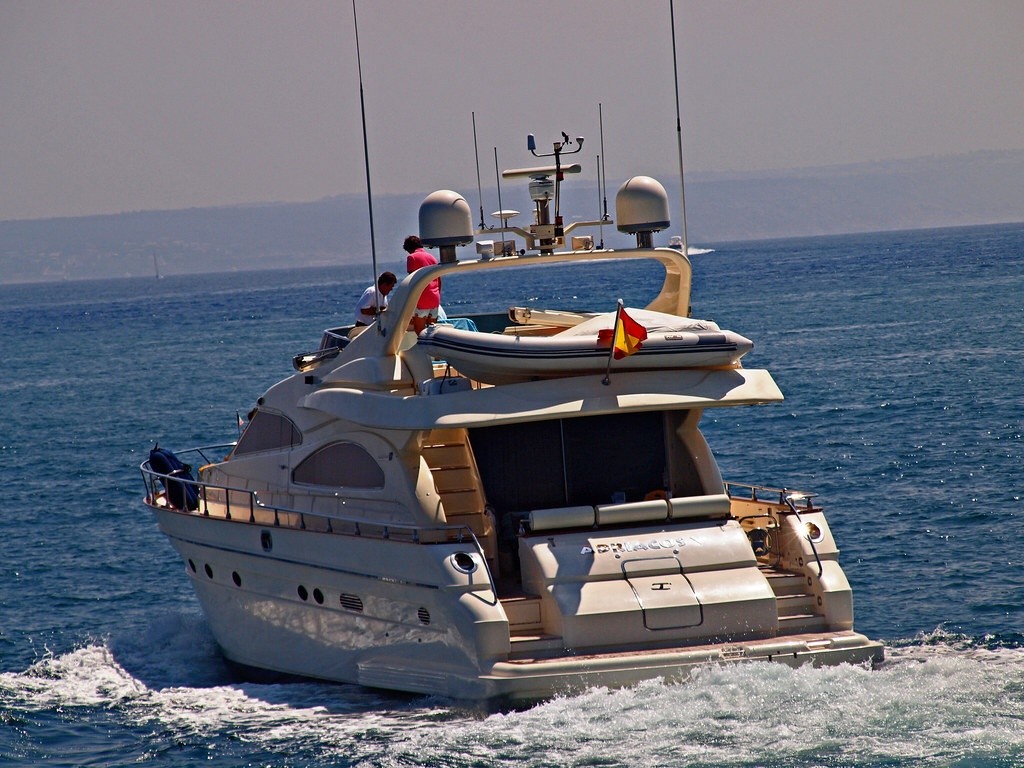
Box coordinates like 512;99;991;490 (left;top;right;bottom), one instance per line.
614;307;647;359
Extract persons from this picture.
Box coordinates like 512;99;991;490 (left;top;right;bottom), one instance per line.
353;272;397;325
403;236;441;333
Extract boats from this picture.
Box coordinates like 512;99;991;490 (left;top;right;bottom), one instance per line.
139;0;886;713
